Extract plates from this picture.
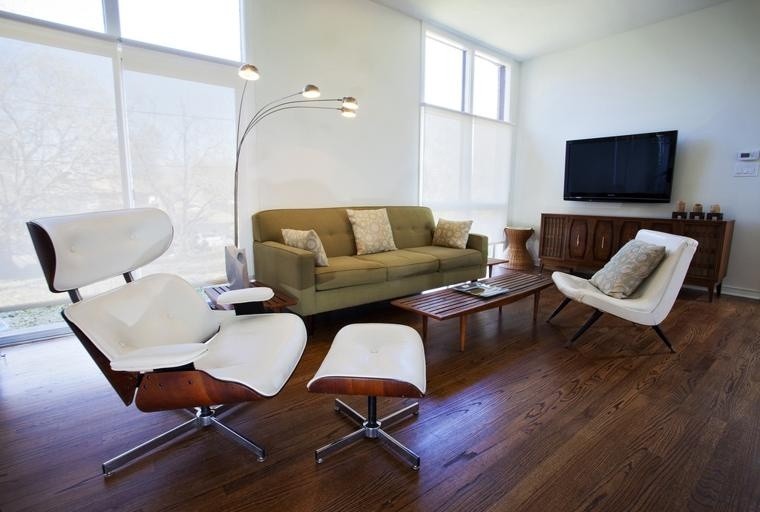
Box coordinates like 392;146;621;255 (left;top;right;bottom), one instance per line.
451;280;508;299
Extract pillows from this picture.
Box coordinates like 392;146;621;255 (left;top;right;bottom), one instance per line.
431;218;473;250
347;208;399;256
587;239;667;300
281;228;335;268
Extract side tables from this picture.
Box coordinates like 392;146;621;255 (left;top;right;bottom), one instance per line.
203;281;298;311
487;257;509;277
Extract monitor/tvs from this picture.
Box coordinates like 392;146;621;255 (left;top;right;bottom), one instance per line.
563;130;679;203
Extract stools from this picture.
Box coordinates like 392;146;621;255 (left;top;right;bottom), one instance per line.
306;321;428;474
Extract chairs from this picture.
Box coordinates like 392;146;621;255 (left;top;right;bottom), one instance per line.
546;227;699;358
25;206;308;476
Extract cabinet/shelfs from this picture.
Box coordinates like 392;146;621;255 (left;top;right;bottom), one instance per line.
537;212;736;302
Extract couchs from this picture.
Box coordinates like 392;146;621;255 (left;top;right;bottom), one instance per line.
251;206;488;333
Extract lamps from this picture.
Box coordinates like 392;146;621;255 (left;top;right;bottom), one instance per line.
234;62;358;247
